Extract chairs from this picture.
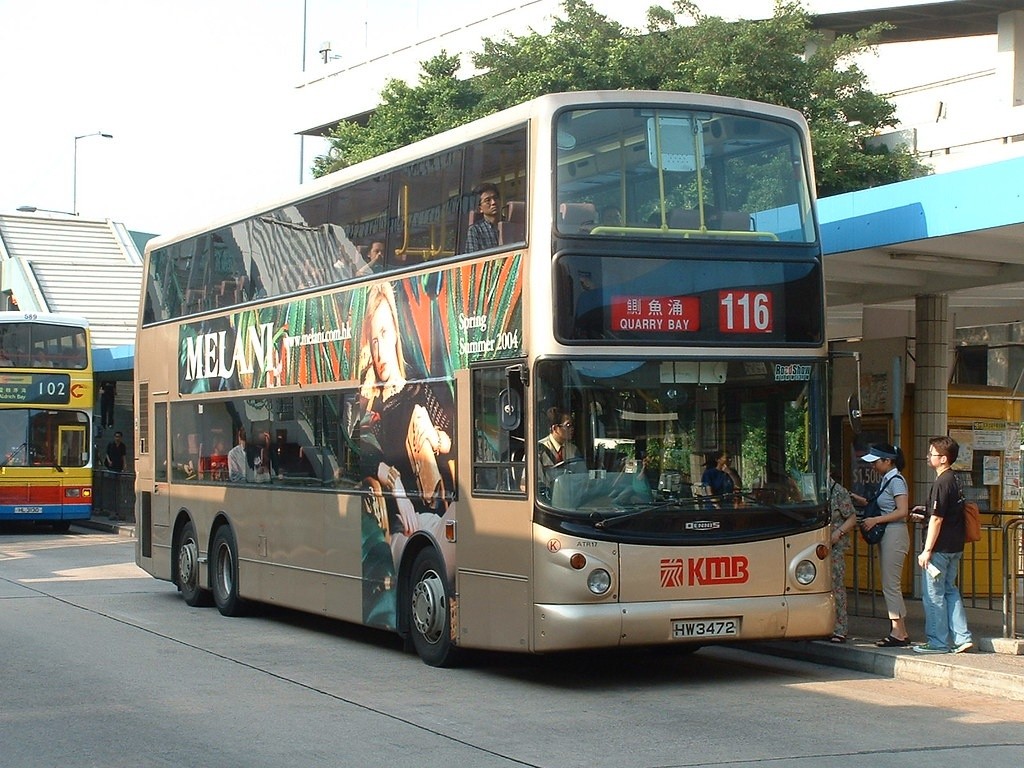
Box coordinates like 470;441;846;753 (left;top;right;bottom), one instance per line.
180;201;751;314
159;466;211;480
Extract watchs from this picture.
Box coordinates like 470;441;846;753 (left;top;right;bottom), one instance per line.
839;528;845;536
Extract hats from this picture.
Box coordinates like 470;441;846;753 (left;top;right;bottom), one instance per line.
861;447;897;463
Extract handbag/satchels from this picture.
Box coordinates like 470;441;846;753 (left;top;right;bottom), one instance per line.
961;501;981;543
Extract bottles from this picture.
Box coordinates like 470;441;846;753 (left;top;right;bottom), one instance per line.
917;554;942;581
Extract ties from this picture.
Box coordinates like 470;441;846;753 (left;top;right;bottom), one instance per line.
557;447;562;462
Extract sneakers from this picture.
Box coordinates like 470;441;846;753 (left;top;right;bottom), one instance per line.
913;644;948;654
951;643;973;652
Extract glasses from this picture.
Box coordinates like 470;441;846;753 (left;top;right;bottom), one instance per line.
478;195;499;205
928;451;944;457
115;435;121;439
550;421;573;430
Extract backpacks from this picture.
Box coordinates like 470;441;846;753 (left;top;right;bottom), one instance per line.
859;475;903;545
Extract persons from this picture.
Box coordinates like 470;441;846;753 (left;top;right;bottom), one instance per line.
251;181;509;298
198;425;284;483
699;450;802;511
0;344;80;367
848;443;911;647
518;407;582;493
343;285;453;535
828;474;858;644
908;438;973;654
99;380;128;515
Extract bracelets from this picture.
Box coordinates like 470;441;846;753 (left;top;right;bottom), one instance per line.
715;504;719;507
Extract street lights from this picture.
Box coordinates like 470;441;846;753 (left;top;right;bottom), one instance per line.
16;132;113;216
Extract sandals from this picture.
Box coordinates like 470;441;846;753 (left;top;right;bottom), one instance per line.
878;635;911;646
830;635;847;643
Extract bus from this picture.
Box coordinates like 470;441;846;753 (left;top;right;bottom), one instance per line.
0;310;104;532
131;91;864;670
